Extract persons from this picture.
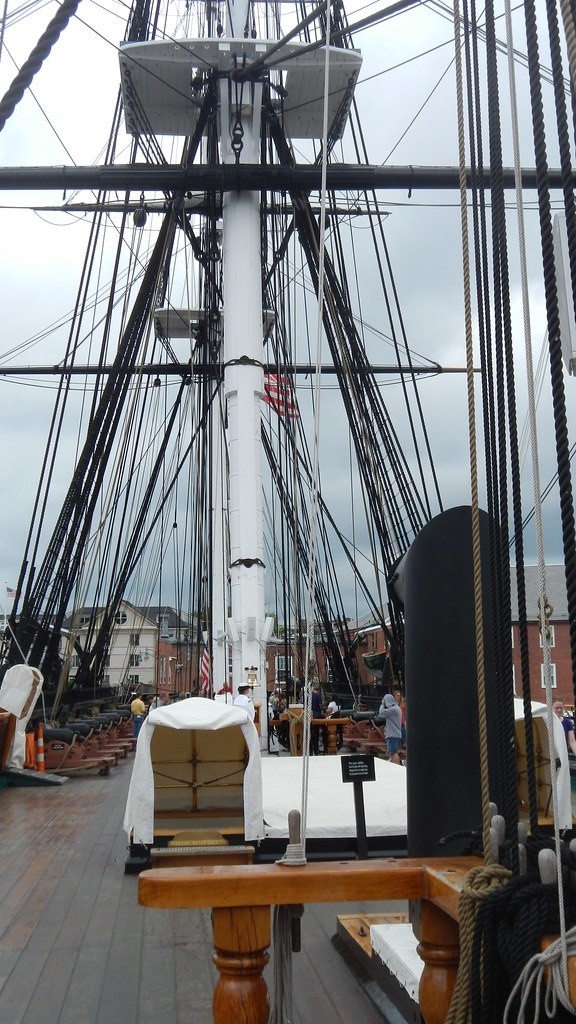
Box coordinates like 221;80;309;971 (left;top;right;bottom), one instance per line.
310;687;324;756
190;680;206;697
552;696;576;754
268;687;287;734
156;692;170;707
219;682;232;695
379;694;402;764
326;695;341;719
130;691;146;744
236;683;255;722
147;695;159;702
393;691;407;749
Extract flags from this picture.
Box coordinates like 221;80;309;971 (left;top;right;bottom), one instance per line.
264;374;300;419
201;639;209;691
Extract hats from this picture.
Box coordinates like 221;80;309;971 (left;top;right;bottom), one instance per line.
239;683;249;687
